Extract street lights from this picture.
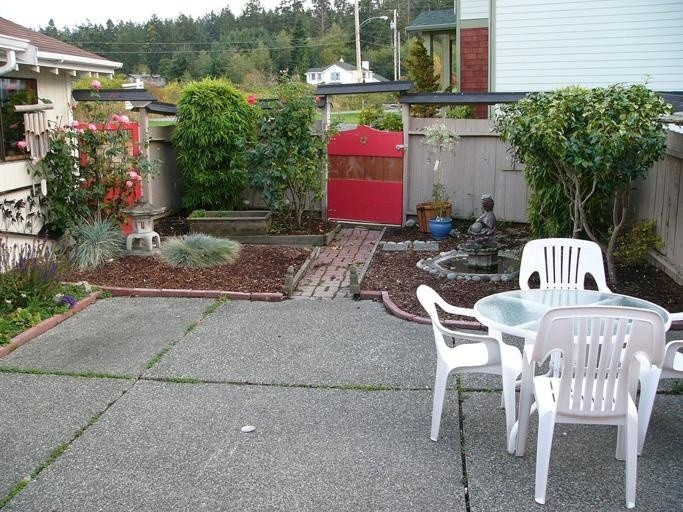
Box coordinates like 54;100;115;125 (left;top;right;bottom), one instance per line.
355;15;390;84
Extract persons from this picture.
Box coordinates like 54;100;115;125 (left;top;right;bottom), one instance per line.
463;193;498;251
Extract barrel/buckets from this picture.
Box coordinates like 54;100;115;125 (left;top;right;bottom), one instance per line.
415;201;451;232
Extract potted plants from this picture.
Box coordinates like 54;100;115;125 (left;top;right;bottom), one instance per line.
415;105;464;239
